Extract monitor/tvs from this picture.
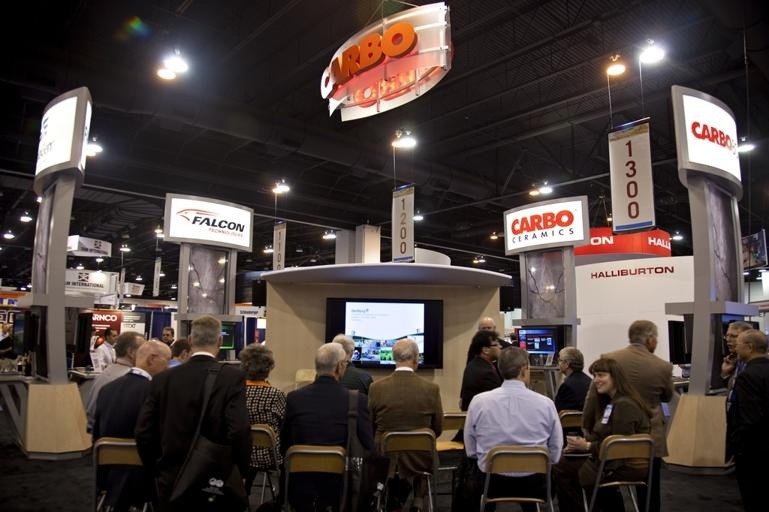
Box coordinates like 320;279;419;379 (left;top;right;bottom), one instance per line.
12;311;28;355
518;327;559;354
326;297;444;369
67;313;93;360
668;320;685;362
730;320;759;330
219;325;235;350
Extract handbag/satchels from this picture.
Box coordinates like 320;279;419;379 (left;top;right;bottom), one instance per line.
170;437;248;511
340;448;390;512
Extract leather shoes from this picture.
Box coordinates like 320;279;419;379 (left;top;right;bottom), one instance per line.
412;497;424;512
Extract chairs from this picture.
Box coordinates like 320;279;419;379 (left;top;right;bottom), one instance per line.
375;428;438;511
436;411;467;511
589;433;655;512
282;444;350;511
558;408;592;511
480;446;554;512
250;423;281;506
90;437;153;512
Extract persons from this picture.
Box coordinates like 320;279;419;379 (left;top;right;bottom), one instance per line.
458;317;519;415
554;347;595;448
566;358;653;512
367;338;443;512
719;321;756;423
161;327;190;368
723;330;767;512
279;342;376;512
85;327;146;433
333;334;374;394
237;342;286;494
462;346;564;512
581;320;674;511
0;323;18;360
91;340;171;512
134;316;252;512
